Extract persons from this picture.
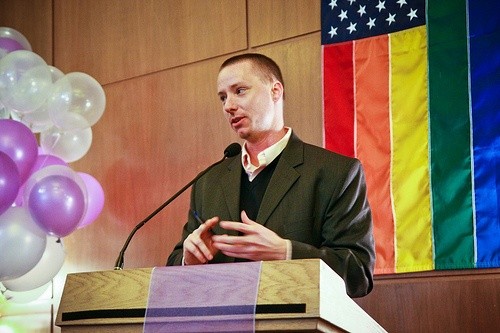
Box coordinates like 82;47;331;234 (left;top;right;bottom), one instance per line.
166;54;376;298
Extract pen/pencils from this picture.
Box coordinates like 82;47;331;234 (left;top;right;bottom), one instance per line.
190;208;218;236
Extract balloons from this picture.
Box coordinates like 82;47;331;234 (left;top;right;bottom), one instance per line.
0;26;106;303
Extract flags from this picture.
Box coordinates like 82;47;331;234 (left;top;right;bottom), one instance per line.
318;0;500;274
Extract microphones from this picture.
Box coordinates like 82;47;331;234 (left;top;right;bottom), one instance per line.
115;143;242;269
